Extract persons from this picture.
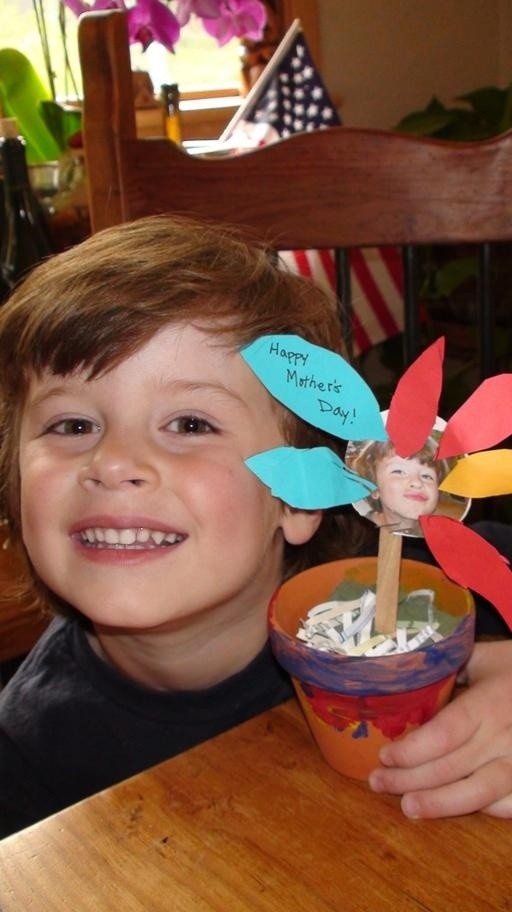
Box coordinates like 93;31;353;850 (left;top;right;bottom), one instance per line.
348;435;450;531
0;216;511;837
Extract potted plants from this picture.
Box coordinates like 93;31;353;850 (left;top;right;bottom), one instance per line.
389;80;512;362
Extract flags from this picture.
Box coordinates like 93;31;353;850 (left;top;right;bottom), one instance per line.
226;29;430;360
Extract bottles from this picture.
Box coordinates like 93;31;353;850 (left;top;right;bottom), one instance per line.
160;82;186;146
1;134;61;299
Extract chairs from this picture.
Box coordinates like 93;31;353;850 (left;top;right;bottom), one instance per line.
76;6;512;558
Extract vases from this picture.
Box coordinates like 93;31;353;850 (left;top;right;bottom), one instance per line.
29;163;69;205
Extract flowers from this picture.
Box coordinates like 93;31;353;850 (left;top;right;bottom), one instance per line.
2;1;268;160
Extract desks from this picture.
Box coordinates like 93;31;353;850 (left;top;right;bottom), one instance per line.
0;689;512;908
